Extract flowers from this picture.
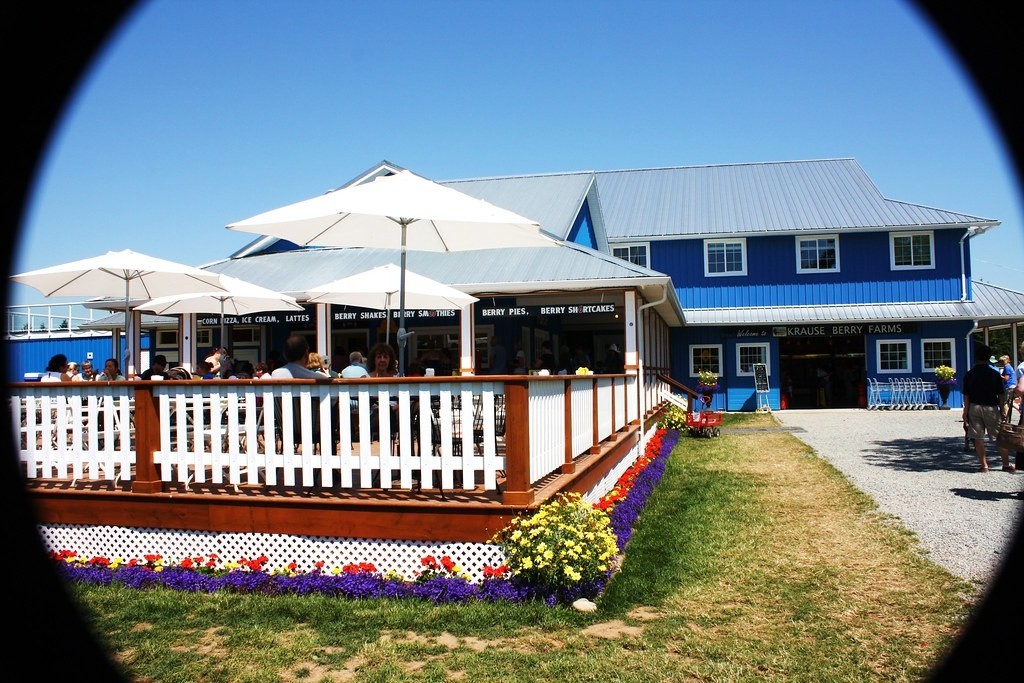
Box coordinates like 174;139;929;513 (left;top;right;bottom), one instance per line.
695;368;721;393
934;365;959;388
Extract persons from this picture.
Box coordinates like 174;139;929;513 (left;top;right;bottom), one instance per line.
963;340;1024;473
36;336;624;448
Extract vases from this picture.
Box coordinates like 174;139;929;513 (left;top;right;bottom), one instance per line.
937;385;951;410
702;391;713;414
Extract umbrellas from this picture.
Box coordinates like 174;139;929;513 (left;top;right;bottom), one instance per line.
8;246;305;383
305;262;481;345
224;168;566;376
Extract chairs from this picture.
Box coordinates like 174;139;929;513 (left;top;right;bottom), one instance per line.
22;395;506;501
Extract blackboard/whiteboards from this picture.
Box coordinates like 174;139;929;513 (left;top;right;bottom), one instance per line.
752;363;770;394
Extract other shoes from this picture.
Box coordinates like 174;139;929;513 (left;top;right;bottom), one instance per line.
978;467;988;472
1002;466;1014;471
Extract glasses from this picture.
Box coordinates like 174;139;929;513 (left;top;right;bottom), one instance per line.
64;365;69;369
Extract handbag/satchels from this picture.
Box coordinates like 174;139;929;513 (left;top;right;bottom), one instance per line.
996;422;1024;452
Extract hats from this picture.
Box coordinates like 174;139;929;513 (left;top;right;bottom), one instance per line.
608;344;620;353
517;351;525;357
989;356;998;363
153;355;168;364
560;345;569;353
